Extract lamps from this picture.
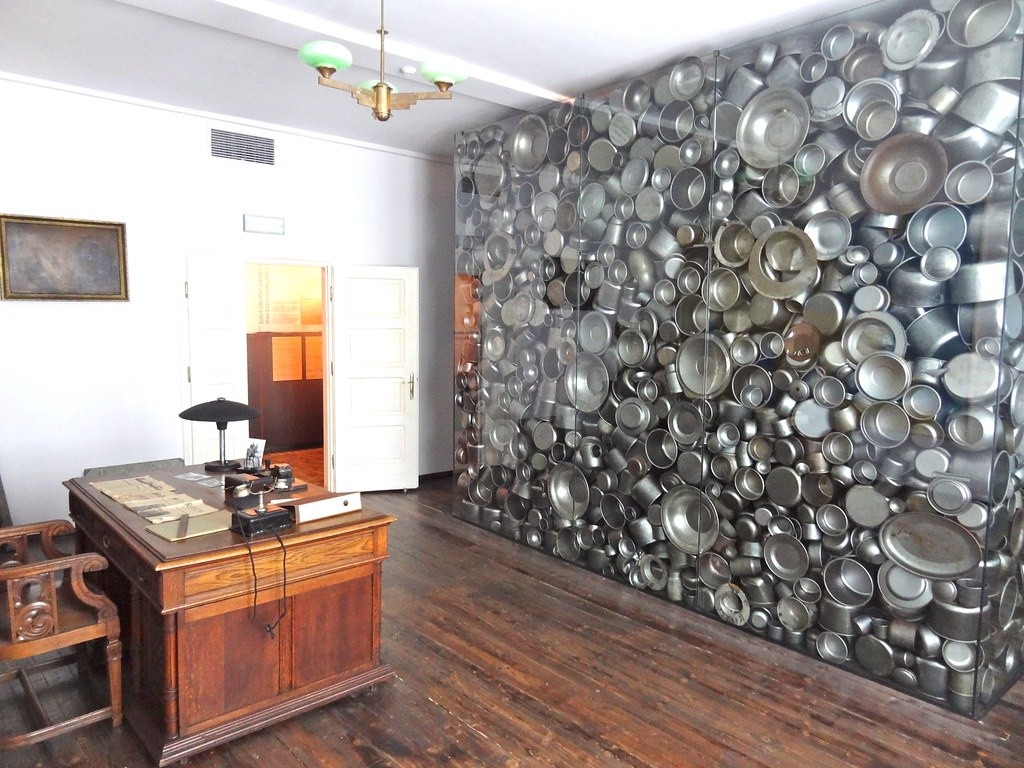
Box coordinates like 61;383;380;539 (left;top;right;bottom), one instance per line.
180;397;261;472
300;0;474;123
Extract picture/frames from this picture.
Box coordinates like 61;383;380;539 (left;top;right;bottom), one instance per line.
1;214;131;302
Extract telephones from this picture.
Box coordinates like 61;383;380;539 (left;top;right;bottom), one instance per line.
231;473;295;538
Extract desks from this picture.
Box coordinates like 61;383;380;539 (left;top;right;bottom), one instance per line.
62;456;397;768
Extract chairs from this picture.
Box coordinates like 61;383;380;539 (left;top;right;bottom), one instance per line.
0;518;124;751
